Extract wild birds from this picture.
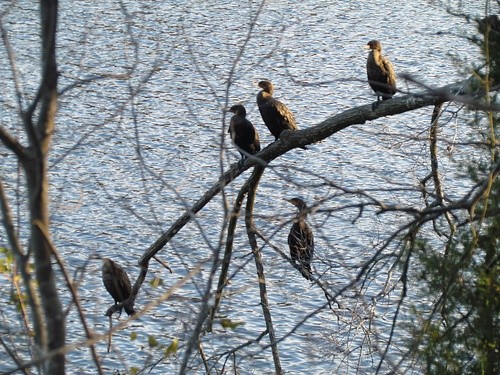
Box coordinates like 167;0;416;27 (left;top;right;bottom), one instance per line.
226;104;260;170
363;40;402;110
282;198;314;281
101;257;136;316
252;80;308;151
